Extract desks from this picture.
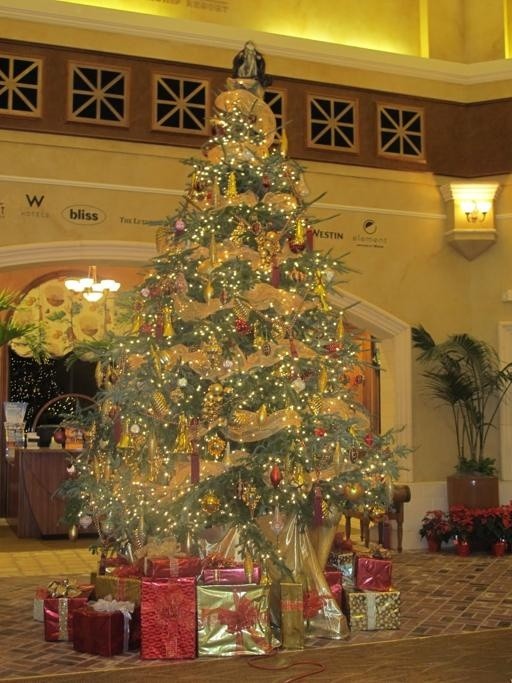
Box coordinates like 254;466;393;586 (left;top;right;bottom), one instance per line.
342;485;411;552
4;445;98;540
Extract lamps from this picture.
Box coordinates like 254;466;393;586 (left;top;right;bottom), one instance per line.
462;201;491;223
63;265;121;302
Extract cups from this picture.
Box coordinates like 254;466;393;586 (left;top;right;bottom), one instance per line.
3;401;29;423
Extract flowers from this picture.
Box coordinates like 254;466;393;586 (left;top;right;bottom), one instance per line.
417;500;512;544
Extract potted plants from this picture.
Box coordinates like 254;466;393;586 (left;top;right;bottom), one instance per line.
408;321;511;507
490;542;506;556
454;543;472;555
428;537;442;552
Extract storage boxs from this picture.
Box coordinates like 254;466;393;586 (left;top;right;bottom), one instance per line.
33;552;403;657
